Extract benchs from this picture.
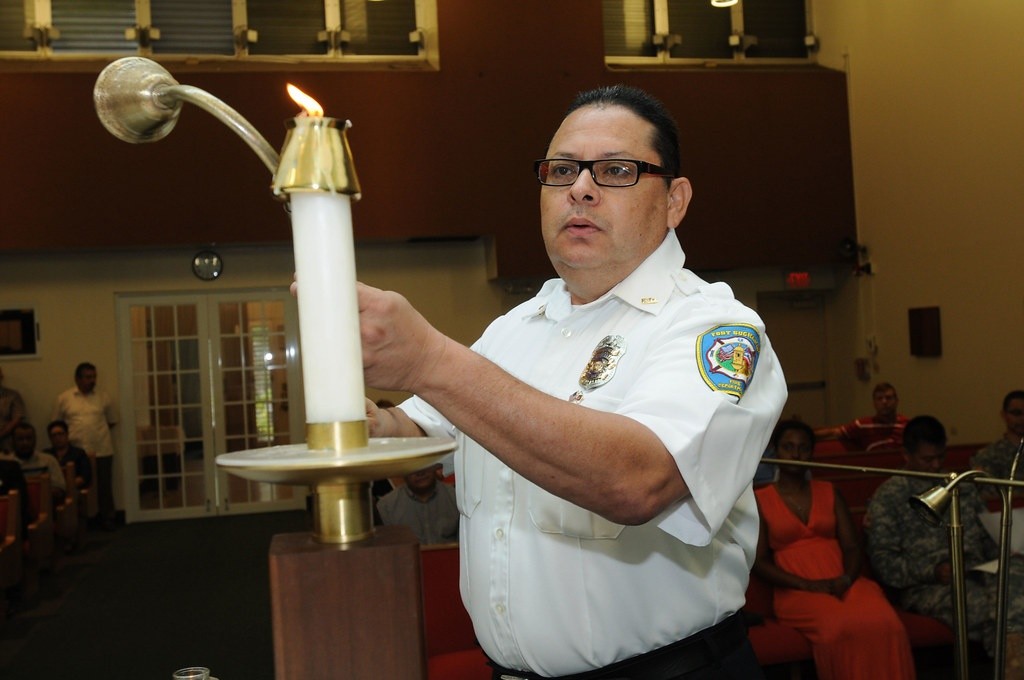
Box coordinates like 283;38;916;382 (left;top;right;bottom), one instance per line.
418;440;1024;680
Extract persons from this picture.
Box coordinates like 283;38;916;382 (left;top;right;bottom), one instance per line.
754;420;917;679
293;83;791;680
863;414;1024;680
0;362;117;583
372;462;459;546
961;390;1024;489
815;381;913;452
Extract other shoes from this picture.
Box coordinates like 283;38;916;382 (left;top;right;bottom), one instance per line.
64;538;74;553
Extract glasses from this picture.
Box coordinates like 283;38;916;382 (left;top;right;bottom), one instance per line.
533;159;674;187
1005;409;1024;415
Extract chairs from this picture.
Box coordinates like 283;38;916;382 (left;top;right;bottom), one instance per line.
0;450;99;590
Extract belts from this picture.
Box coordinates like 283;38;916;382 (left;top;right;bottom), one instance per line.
594;614;747;680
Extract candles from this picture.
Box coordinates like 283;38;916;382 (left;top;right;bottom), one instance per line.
272;83;367;449
